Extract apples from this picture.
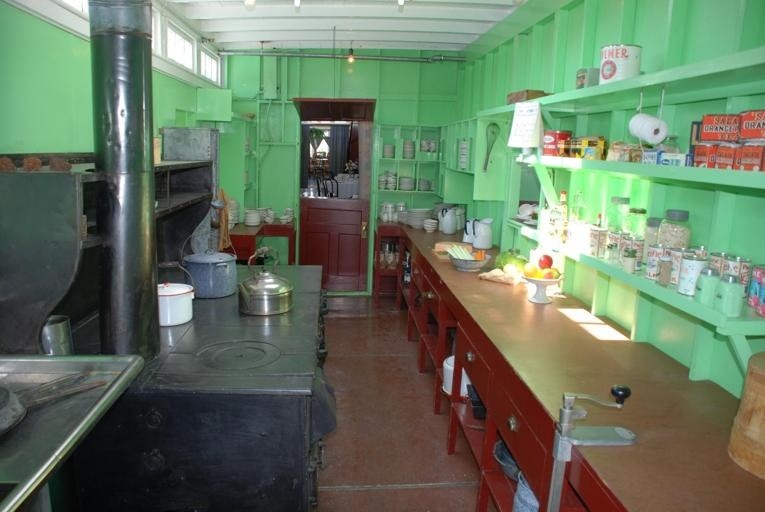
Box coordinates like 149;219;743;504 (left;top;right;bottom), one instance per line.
524;255;561;280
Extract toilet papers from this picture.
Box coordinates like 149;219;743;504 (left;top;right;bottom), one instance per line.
629;114;667;146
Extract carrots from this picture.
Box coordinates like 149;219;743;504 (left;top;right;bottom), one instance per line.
474;250;486;260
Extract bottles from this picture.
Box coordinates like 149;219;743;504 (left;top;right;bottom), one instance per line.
37;315;74;356
557;190;569;217
603;195;693;272
692;266;746;319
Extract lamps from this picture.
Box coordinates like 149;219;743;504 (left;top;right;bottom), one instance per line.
348;40;355;64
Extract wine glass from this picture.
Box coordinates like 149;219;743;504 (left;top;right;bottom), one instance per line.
522;274;562;305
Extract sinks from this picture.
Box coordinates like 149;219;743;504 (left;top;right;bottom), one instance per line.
0;482;17;506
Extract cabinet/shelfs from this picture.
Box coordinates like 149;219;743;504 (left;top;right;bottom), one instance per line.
375;123;438;194
1;162;214;358
373;224;403;309
452;300;624;512
507;43;765;414
399;229;457;458
220;118;258;221
438;116;506;207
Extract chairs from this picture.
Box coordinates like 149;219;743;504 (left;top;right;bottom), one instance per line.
312;166;339;198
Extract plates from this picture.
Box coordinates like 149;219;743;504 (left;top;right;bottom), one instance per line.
399;177;414;190
419;179;431;191
383;143;395;159
229;199;241;226
403;140;416;159
243;209;260;227
407;207;432;230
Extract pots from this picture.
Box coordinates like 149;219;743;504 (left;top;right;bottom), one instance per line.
0;368;108;435
181;232;240;300
157;261;197;328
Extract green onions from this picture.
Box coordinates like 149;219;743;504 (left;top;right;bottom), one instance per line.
445;243;474;260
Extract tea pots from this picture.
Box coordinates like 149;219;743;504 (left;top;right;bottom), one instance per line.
472;216;496;251
236;245;295;315
462;217;480;243
441;208;458;234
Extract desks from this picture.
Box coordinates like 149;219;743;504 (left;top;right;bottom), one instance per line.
226;218;296;264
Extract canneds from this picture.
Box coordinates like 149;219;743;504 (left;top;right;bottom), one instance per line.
694;111;765;171
598;44;642;85
590;198;764;319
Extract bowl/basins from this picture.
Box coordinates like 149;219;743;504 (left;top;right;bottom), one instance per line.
459;142;468;169
449;252;491;273
489;439;540;511
378;168;396;190
260;207;274;223
279;205;294;225
440;356;472;399
423;217;438;232
